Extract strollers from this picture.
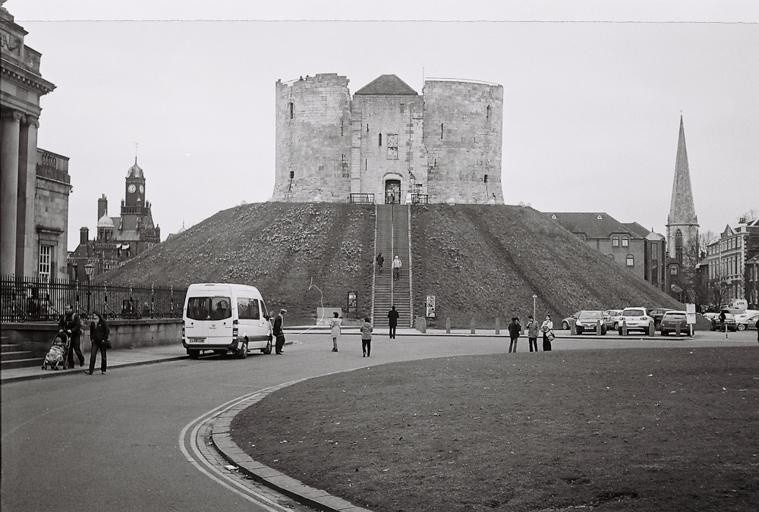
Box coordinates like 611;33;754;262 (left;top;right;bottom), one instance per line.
41;330;71;371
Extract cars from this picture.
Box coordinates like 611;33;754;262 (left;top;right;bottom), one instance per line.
703;308;759;331
701;298;748;312
561;306;695;338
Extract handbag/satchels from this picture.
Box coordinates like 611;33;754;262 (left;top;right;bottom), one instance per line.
104;341;112;350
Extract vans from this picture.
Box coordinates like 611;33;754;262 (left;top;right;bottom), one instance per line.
183;282;275;358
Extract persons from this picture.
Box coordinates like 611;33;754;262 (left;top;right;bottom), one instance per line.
53;304;109;375
377;252;384;272
526;315;539;352
540;314;553;351
719;310;726;333
360;318;373;357
272;309;288;354
328;312;341;352
508;317;521;353
387;306;399;339
392;255;402;280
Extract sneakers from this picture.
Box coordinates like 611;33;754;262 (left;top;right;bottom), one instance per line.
84;371;92;375
80;356;85;367
276;350;284;355
331;348;339;352
68;364;75;369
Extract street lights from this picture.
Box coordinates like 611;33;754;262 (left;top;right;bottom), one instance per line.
82;259;93;318
531;292;539;320
